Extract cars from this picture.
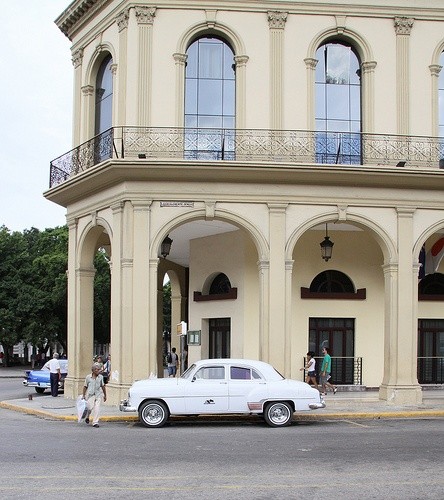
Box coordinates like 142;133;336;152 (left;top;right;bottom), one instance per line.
119;358;326;428
22;359;108;393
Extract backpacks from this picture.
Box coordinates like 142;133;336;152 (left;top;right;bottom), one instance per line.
167;353;172;362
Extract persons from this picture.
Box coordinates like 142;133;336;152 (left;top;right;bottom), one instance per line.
319;347;338;395
300;351;318;389
0;352;7;367
166;347;178;378
46;353;62;398
31;349;46;369
180;346;187;371
93;354;112;383
81;365;107;427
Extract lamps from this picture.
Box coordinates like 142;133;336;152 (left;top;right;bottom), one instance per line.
320;224;334;263
160;234;173;258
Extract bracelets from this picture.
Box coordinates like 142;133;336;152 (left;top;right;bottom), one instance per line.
323;370;326;372
304;367;306;369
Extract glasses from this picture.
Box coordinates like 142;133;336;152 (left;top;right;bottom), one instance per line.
322;349;324;351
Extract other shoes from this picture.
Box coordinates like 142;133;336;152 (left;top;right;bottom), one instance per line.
334;387;338;394
85;418;89;423
320;392;327;395
94;424;99;428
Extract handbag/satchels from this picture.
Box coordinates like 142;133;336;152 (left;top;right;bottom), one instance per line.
313;372;317;376
